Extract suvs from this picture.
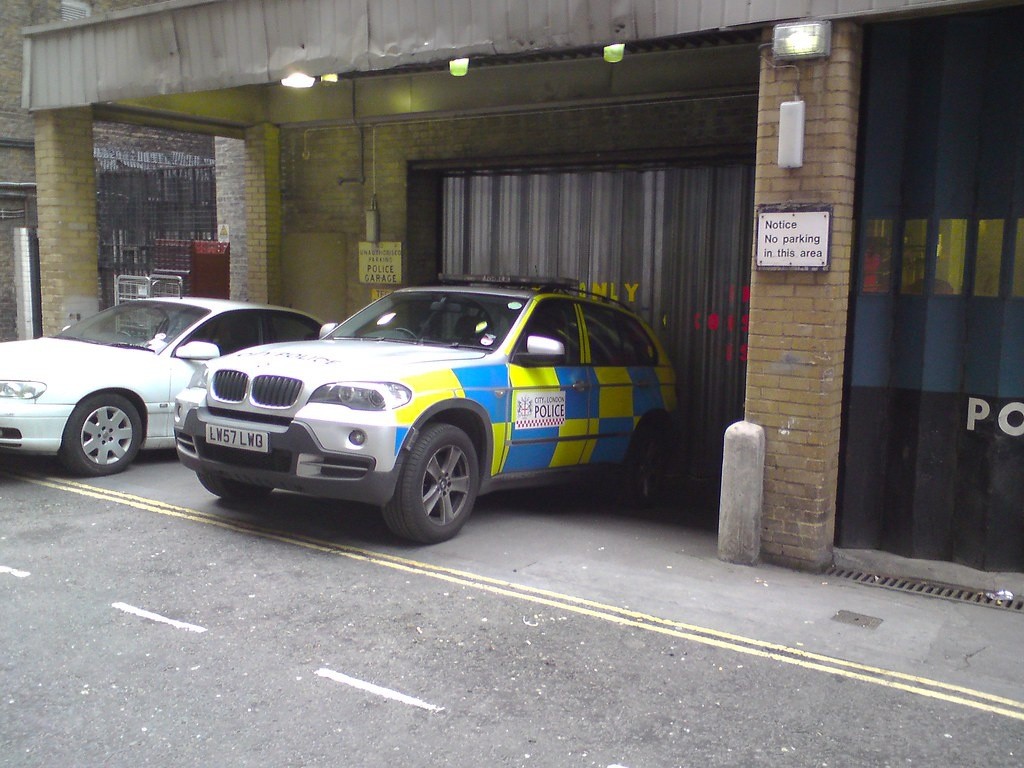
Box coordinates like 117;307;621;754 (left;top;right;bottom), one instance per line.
173;275;689;548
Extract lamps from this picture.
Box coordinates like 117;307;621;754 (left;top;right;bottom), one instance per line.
754;20;833;169
281;71;339;88
449;58;469;76
366;128;382;243
602;43;625;64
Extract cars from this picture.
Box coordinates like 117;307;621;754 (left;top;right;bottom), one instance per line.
0;296;332;476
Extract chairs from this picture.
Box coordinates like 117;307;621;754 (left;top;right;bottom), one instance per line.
454;305;491;345
508;310;560;354
